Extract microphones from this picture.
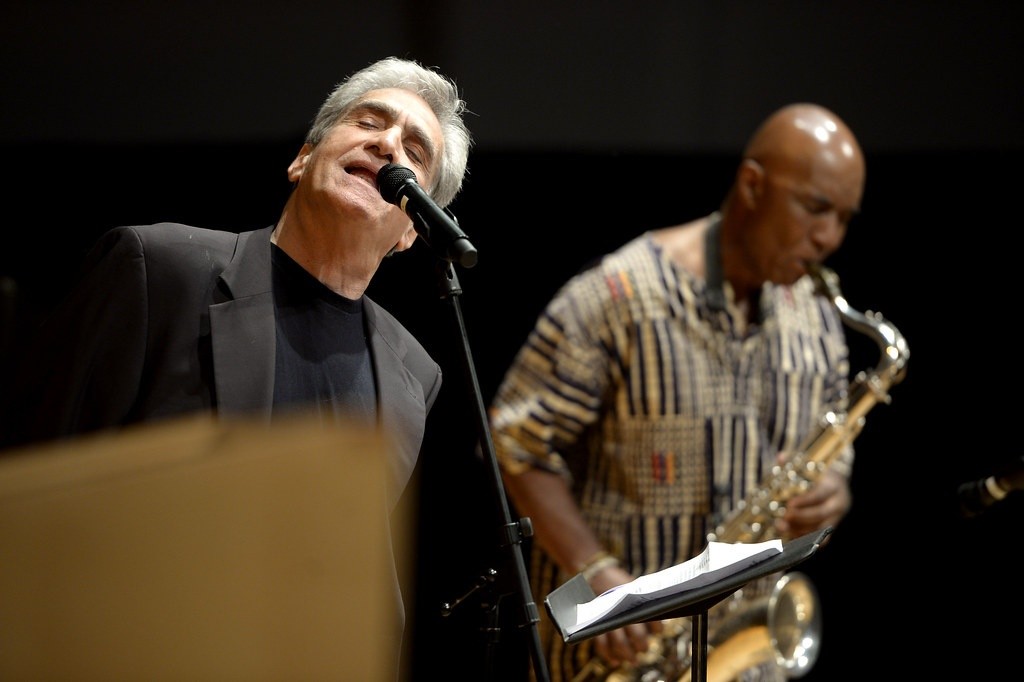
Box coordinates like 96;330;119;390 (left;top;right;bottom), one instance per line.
377;163;477;268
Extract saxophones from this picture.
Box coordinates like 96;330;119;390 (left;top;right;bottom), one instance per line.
551;250;925;682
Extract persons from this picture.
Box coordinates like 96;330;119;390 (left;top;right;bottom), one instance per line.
58;53;472;439
477;99;866;682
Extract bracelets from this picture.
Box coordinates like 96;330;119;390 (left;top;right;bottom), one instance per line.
574;551;621;583
